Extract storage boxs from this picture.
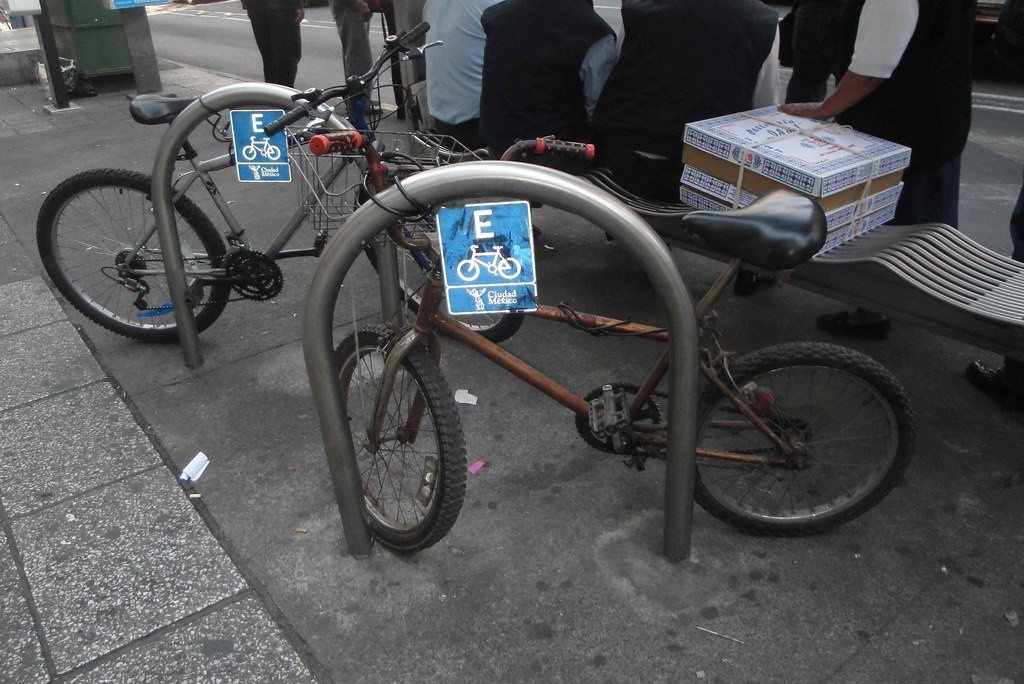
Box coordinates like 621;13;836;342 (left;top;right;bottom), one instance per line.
679;105;912;258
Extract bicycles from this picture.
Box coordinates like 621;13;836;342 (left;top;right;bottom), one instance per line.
307;128;918;561
31;20;537;346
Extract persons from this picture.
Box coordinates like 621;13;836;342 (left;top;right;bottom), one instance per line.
777;0;978;342
589;0;780;297
241;0;305;89
328;0;383;117
480;0;618;174
966;177;1024;411
421;0;505;153
779;0;865;105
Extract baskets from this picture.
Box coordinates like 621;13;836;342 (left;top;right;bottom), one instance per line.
285;126;486;249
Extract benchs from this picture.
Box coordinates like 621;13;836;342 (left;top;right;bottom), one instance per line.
565;163;1024;361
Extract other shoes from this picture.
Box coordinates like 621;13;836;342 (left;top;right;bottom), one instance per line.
734;267;779;298
815;308;893;341
966;358;1024;415
